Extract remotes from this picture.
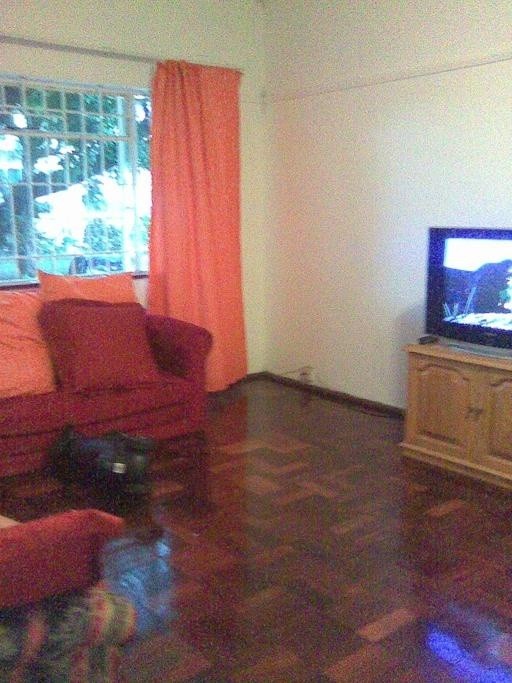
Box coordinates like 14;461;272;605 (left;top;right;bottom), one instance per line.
420;336;438;344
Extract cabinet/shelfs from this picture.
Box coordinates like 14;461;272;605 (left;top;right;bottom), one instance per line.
398;333;512;500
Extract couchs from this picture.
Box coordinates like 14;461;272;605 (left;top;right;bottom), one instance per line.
0;311;212;490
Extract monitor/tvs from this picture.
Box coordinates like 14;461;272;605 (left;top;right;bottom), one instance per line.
425;226;512;360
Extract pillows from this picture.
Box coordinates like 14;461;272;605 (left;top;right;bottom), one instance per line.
0;270;154;406
